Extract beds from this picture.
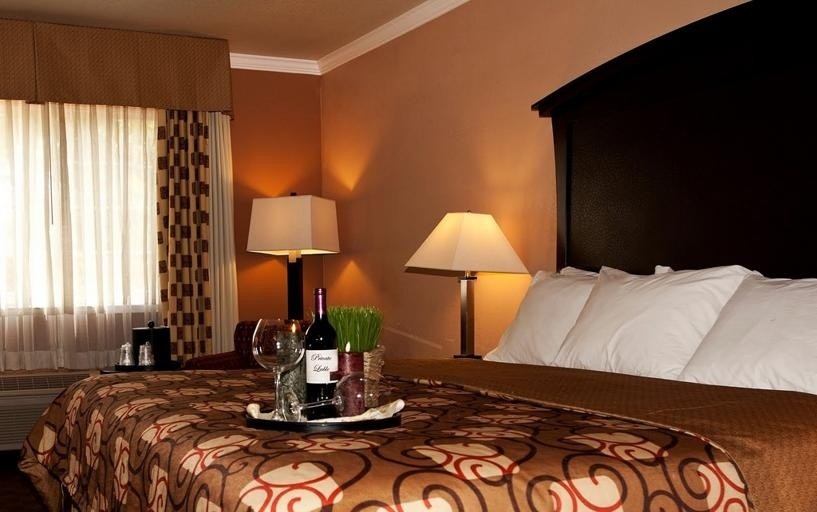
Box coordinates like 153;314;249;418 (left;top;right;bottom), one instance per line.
21;2;817;512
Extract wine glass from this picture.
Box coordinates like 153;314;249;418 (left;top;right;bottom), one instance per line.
252;317;304;422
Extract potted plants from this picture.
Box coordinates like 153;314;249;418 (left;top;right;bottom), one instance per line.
309;305;385;415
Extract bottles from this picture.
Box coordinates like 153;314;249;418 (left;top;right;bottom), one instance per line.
304;287;339;403
139;345;154;366
119;344;136;365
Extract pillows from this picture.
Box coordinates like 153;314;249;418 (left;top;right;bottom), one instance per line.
681;273;817;394
553;265;751;385
482;264;599;366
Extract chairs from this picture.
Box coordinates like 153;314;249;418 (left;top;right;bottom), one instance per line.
185;319;310;370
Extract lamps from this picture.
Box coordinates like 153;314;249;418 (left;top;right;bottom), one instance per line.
246;191;342;322
404;211;530;359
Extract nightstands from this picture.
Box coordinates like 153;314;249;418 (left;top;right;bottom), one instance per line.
99;363;184;374
